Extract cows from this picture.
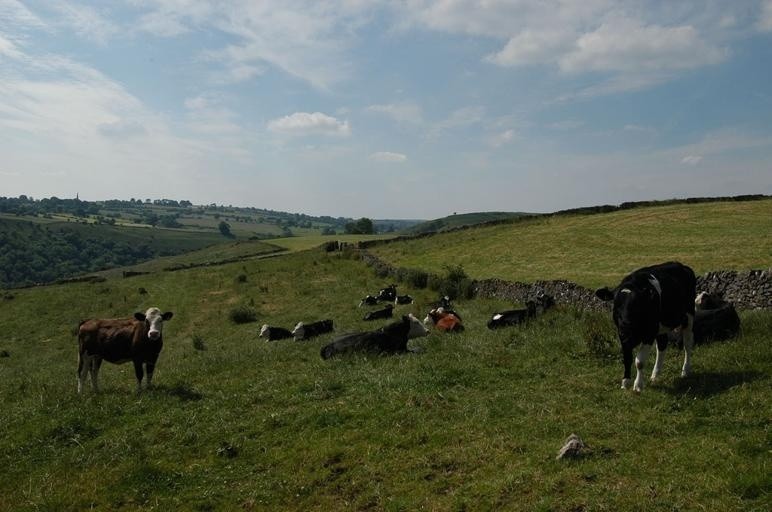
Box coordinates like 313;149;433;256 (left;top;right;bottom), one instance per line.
670;290;740;349
358;283;413;308
76;306;174;396
487;300;537;330
595;261;697;393
259;324;292;343
292;319;333;341
423;294;465;333
363;303;393;322
320;313;431;361
537;293;555;309
